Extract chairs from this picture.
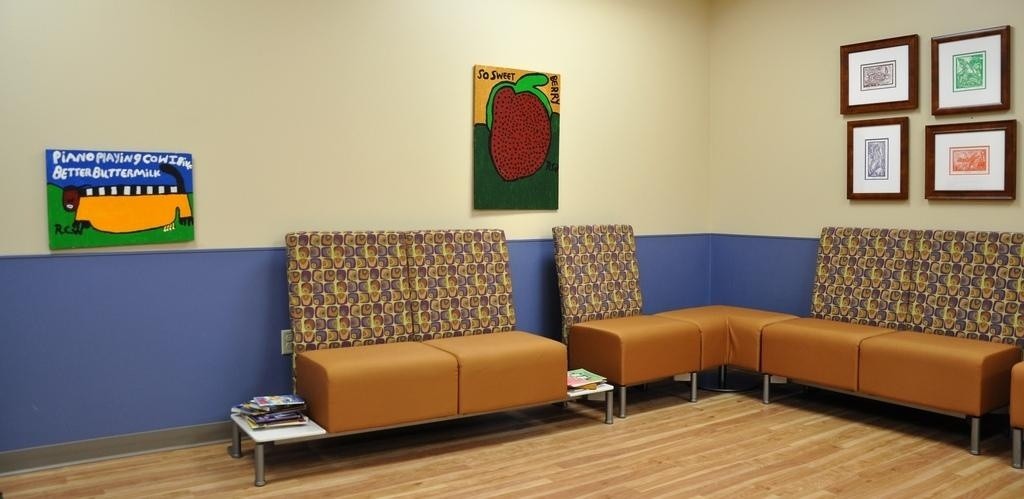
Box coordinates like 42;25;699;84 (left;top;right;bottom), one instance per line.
549;220;703;417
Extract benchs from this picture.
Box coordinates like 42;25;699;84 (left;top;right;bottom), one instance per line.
225;223;619;488
758;220;1024;456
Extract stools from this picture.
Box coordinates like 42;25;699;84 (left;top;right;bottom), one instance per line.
1006;353;1024;472
647;295;798;406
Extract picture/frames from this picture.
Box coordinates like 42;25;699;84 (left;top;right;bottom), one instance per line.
928;20;1015;116
835;32;922;116
843;116;913;201
924;116;1019;201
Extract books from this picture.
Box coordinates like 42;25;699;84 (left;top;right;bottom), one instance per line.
568;367;606;392
230;394;309;430
